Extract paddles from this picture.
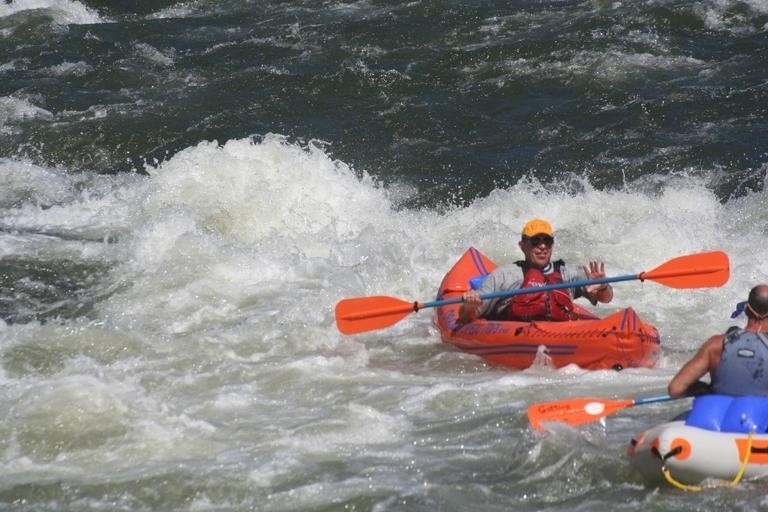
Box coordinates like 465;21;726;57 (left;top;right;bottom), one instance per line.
335;251;730;335
526;395;695;431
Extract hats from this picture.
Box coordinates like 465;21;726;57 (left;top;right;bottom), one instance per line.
523;219;554;239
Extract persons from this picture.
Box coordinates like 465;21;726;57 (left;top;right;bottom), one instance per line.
455;218;614;321
668;284;768;399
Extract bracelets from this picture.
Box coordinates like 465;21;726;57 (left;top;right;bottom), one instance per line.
598;282;609;291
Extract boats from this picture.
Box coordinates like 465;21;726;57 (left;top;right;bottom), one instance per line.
628;395;767;490
434;246;665;375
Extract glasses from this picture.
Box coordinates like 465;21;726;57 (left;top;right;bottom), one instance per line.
522;235;554;247
